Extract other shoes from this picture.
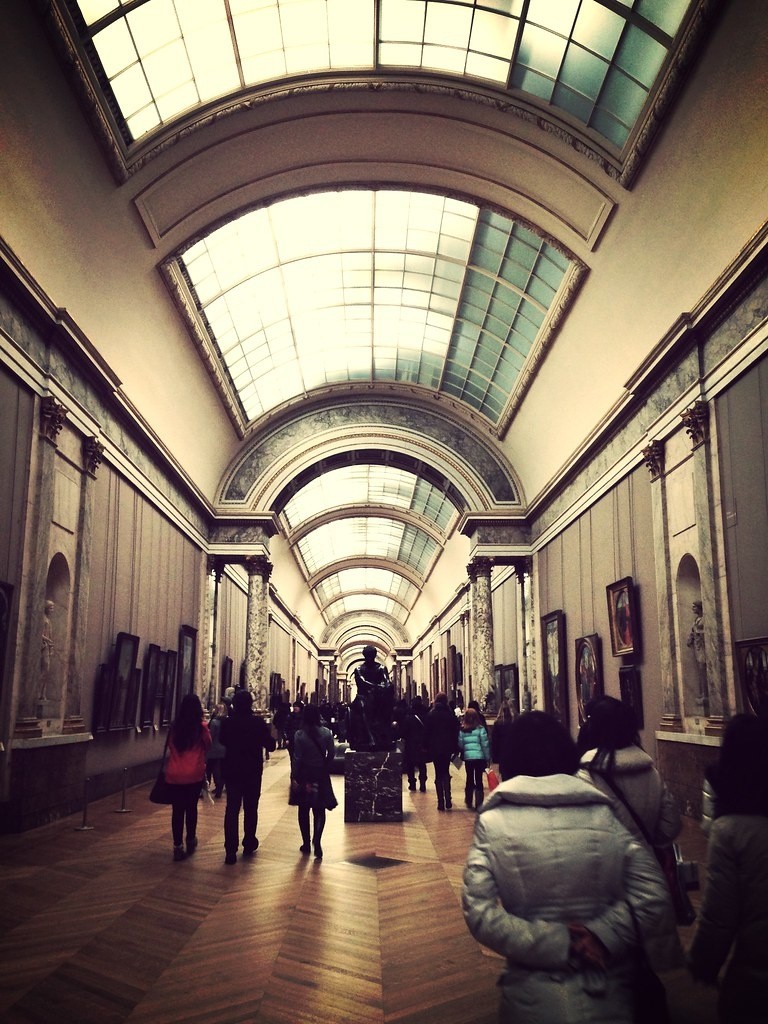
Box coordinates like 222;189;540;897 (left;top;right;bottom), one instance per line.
226;854;237;864
465;797;472;807
313;840;322;858
300;845;310;852
215;792;221;798
244;838;259;854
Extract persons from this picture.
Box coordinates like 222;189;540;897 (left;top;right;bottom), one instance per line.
419;692;461;810
687;600;709;697
690;713;768;1024
580;695;684;970
393;696;428;792
288;703;338;857
461;711;677;1024
350;646;389;746
165;693;212;860
272;698;350;750
489;701;519;781
456;708;490;809
206;687;235;798
218;691;276;864
37;600;55;700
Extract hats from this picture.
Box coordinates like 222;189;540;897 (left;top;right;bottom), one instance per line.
220;696;232;705
703;715;768;813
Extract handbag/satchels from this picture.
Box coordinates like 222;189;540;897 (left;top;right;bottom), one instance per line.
676;860;701;890
654;845;697;926
620;899;670;1024
484;767;499;791
149;771;173;804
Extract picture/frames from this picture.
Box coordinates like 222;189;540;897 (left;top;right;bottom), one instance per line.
222;657;233;696
540;609;569;733
270;672;286;695
575;632;602;729
606;575;640;658
495;662;518;715
92;624;199;733
619;665;644;729
430;645;463;703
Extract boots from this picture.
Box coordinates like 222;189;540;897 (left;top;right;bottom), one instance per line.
186;836;198;853
173;842;185;860
419;775;428;792
434;779;445;811
444;776;453;808
408;778;416;790
